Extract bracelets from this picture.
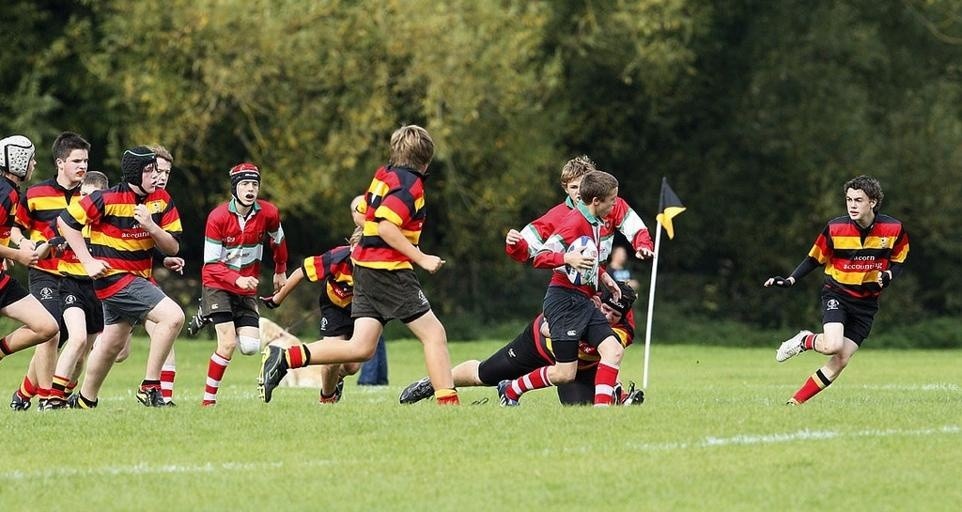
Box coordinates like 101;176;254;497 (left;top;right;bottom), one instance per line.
17;237;24;247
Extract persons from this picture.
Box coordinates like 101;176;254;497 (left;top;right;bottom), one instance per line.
260;124;488;409
764;175;910;413
46;171;116;412
0;135;58;362
400;282;637;405
56;148;187;410
351;196;389;387
91;146;184;408
11;131;97;411
506;156;656;406
496;170;624;410
202;163;287;409
603;247;636;333
259;227;366;404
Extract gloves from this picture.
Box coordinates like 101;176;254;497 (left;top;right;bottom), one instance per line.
770;276;795;289
260;297;280;309
881;272;890;288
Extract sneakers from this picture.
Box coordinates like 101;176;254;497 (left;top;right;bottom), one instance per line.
399;376;434;406
320;380;343;403
257;345;288;403
135;381;166;407
37;394;98;411
188;298;209;334
498;380;520;407
10;392;31;411
620;383;644;406
776;329;813;362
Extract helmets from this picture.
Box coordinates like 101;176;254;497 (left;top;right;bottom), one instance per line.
229;163;260;185
598;281;636;322
0;136;35;178
121;147;156;186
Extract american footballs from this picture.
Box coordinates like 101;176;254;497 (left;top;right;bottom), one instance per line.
565;235;599;287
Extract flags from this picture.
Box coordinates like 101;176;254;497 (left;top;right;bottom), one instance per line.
655;178;685;240
